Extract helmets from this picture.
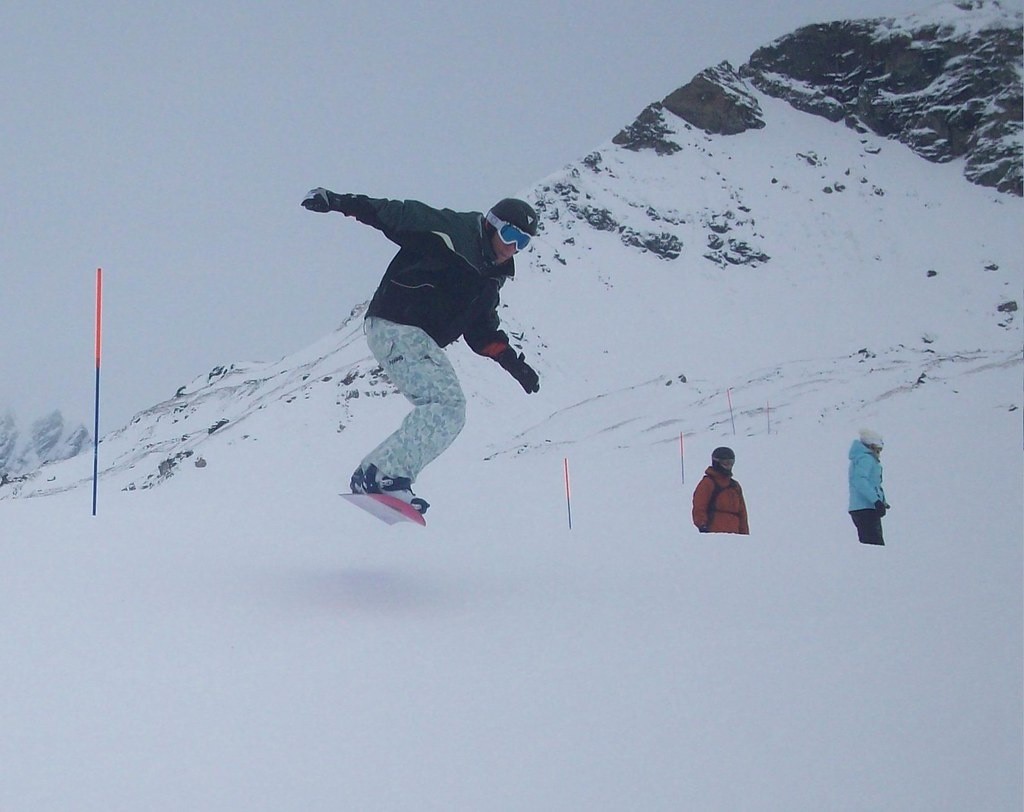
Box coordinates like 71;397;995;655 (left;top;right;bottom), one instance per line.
711;447;735;477
860;431;883;451
487;198;538;254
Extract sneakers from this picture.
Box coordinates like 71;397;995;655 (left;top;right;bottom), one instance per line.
350;462;430;514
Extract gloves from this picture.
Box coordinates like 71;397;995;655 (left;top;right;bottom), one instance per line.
493;347;540;394
300;186;346;213
875;500;886;517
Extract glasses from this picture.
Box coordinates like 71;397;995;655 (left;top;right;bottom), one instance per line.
498;221;532;251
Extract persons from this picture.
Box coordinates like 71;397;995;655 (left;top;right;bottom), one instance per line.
848;428;891;548
691;446;750;534
300;185;540;526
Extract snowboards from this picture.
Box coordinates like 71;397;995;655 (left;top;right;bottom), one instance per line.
339;489;428;529
